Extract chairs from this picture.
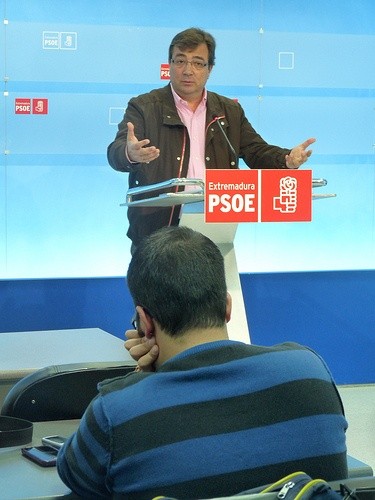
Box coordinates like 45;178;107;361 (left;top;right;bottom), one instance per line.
0;361;139;423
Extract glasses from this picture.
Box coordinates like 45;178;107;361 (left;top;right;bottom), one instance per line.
132;320;138;329
170;58;210;70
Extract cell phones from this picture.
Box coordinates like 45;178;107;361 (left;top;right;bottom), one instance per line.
21;444;59;467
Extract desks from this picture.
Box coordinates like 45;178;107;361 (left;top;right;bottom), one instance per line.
0;327;375;500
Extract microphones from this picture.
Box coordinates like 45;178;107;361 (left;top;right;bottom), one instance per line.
212;112;239;169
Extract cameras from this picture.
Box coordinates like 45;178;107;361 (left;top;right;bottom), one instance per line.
41;434;67;451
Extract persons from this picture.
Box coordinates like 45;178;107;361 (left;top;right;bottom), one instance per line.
56;225;348;500
106;27;316;258
